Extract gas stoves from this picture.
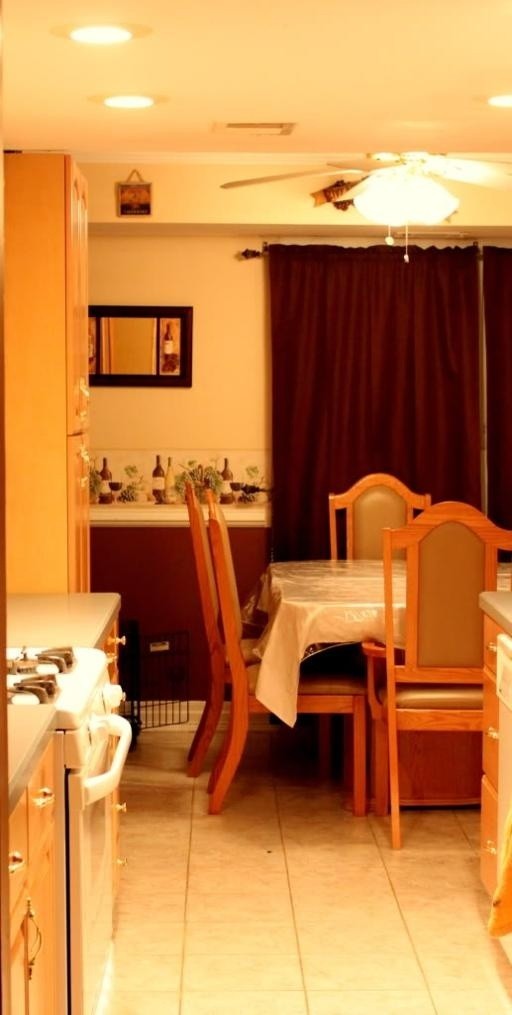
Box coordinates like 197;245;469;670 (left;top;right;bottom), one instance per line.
7;645;127;732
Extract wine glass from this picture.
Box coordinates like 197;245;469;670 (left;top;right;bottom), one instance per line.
108;479;123;506
230;482;244;505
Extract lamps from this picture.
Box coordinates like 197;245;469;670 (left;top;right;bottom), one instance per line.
350;167;461;225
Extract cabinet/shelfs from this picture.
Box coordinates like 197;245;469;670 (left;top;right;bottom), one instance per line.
3;150;93;599
10;743;65;1015
476;613;499;902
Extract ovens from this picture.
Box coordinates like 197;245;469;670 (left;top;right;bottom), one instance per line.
55;713;136;1015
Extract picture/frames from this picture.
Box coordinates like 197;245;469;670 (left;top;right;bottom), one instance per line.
116;180;152;217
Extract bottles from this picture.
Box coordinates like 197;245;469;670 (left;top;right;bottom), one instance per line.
219;457;234;504
162;322;175;362
164;456;177;505
152;455;165;505
98;458;113;504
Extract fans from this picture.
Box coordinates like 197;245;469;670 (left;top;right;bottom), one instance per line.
216;151;509;209
